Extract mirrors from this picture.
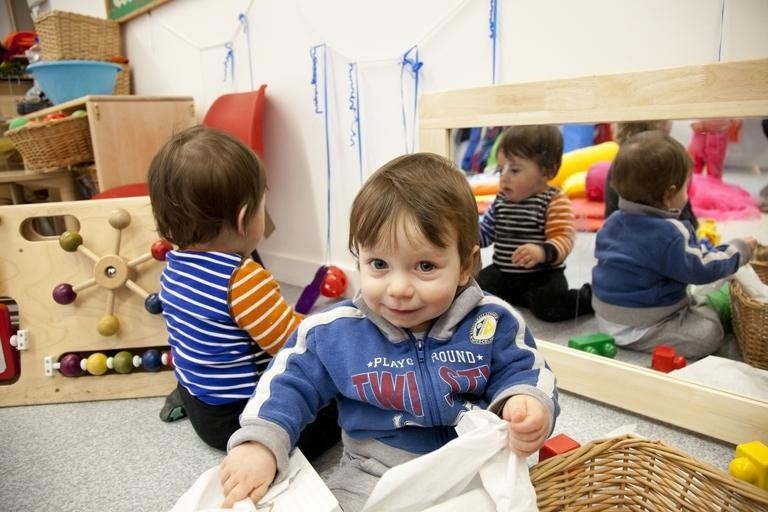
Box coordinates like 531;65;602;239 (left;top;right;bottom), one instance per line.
414;57;768;456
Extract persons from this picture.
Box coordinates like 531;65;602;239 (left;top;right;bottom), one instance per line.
218;150;560;510
475;124;593;322
147;125;343;465
590;130;758;360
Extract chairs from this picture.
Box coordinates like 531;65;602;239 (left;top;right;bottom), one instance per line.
93;84;266;199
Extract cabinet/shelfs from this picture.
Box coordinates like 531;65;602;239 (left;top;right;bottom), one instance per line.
0;95;194;203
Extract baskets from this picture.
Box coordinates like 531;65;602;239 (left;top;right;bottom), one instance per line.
113;61;130;96
527;434;768;512
727;250;768;373
3;113;93;167
34;10;121;61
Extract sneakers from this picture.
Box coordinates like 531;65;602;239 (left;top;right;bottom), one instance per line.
158;389;186;423
